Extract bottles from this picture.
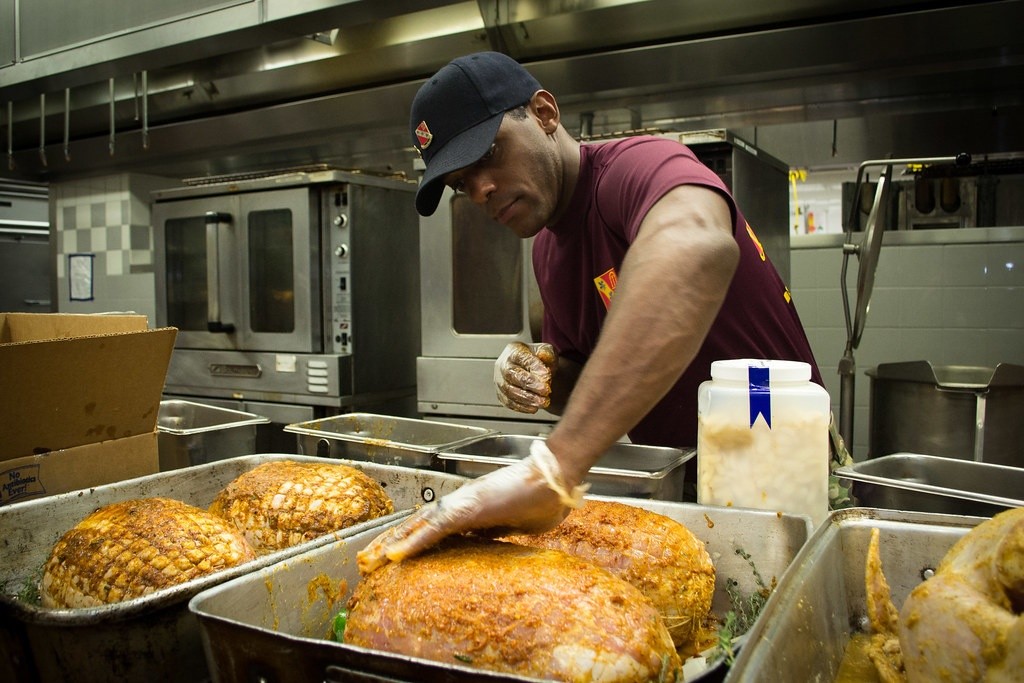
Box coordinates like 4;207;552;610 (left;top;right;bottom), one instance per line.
695;360;832;528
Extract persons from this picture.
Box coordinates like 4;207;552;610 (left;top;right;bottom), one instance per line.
355;51;857;575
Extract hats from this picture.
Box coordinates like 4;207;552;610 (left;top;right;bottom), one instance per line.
409;52;545;217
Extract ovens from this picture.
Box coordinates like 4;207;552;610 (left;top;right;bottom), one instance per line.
417;131;789;419
148;171;423;408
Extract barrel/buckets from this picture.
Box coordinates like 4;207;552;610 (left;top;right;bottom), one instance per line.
865;362;1024;467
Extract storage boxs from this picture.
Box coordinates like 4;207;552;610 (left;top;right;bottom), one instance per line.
0;312;178;506
